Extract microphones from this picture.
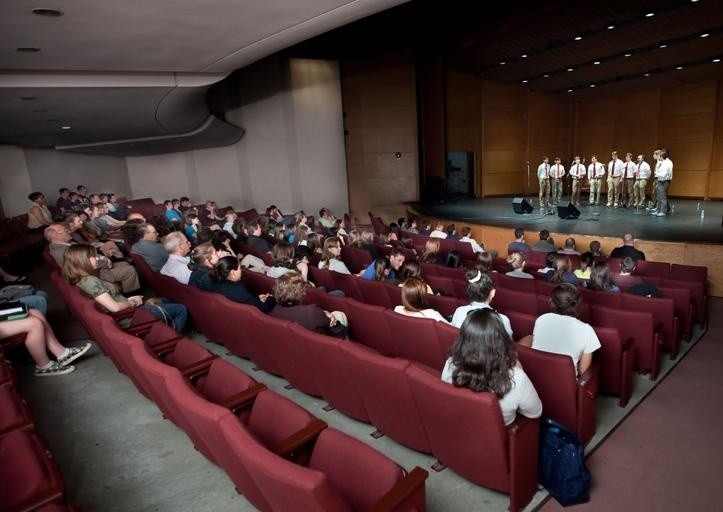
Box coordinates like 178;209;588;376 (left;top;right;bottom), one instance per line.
526;160;530;165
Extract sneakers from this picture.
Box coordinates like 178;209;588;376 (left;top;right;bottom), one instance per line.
645;203;672;216
570;201;580;206
587;202;601;207
539;199;561;207
33;342;92;376
606;203;644;208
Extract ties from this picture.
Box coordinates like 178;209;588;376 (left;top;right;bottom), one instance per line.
557;164;560;178
612;161;615;175
624;164;628;177
577;164;579;175
545;164;547;178
637;164;640;177
593;163;596;176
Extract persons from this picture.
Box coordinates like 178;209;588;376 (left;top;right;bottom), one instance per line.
606;150;673;217
440;307;543;426
566;154;605;207
1;186;664;377
537;155;565;208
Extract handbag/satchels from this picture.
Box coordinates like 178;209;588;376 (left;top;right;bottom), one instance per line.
538;418;590;506
0;285;35;300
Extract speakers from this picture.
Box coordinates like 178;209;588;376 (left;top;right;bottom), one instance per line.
446;150;473;199
557;200;580;219
512;197;534;213
